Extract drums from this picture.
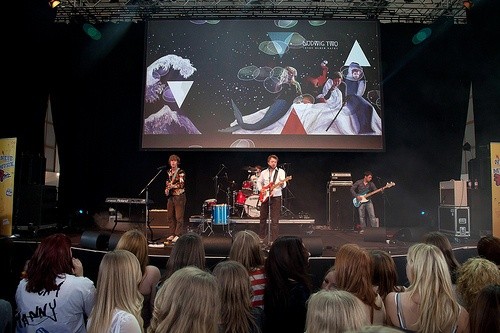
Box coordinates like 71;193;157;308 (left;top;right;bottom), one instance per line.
205;198;218;210
235;190;255;205
242;181;255;189
244;195;262;219
211;204;229;226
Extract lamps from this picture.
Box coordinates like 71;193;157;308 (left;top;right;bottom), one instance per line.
464;0;474;7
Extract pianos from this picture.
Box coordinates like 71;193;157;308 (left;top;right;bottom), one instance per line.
105;198;154;242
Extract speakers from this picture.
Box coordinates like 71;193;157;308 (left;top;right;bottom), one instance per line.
440;181;467;206
304;238;322;256
203;236;233;256
326;181;354;230
109;234;122;250
36;225;56;238
16;226;34;239
392;227;422;242
81;231;108;250
364;227;386;242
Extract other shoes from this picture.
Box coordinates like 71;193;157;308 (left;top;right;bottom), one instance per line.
167;235;174;241
172;236;179;243
360;229;364;235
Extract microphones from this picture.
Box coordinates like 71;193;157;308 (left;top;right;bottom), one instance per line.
157;166;167;169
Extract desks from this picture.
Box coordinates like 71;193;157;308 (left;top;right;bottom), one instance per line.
48;0;61;8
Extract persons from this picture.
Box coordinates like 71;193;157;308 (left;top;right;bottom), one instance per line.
350;171;382;234
15;231;500;333
165;155;186;242
250;155;288;245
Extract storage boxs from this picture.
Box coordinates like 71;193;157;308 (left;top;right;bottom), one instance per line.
438;207;470;238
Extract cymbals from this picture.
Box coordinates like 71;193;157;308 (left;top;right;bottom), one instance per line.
240;167;262;173
279;162;292;167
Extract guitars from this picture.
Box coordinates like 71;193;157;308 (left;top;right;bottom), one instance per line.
258;175;293;203
164;175;178;199
352;181;396;209
